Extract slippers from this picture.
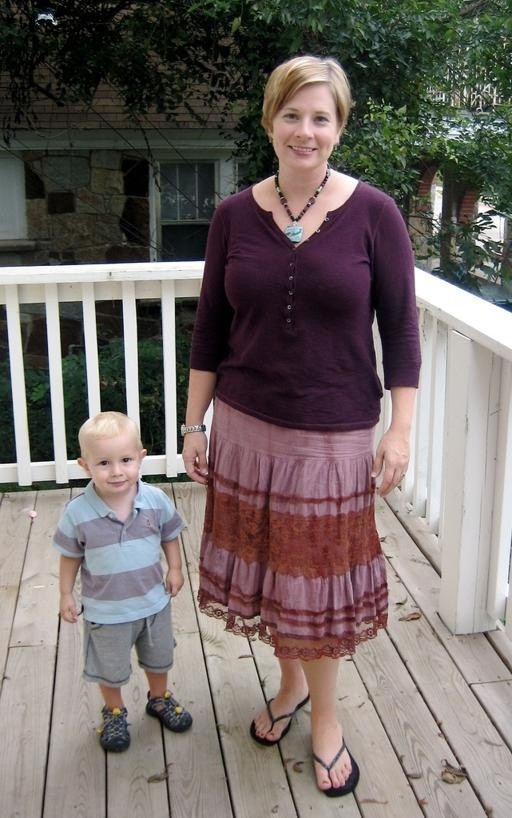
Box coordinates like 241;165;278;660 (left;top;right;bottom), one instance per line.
312;735;360;797
248;689;310;747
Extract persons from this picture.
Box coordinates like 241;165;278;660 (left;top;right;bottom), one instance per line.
51;410;195;754
178;53;426;798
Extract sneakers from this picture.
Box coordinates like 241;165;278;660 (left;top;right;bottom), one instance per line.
145;688;193;733
96;704;132;752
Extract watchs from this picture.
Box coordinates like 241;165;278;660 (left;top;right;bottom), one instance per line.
180;422;207;437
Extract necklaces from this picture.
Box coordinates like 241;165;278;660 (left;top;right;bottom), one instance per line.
274;161;332;246
